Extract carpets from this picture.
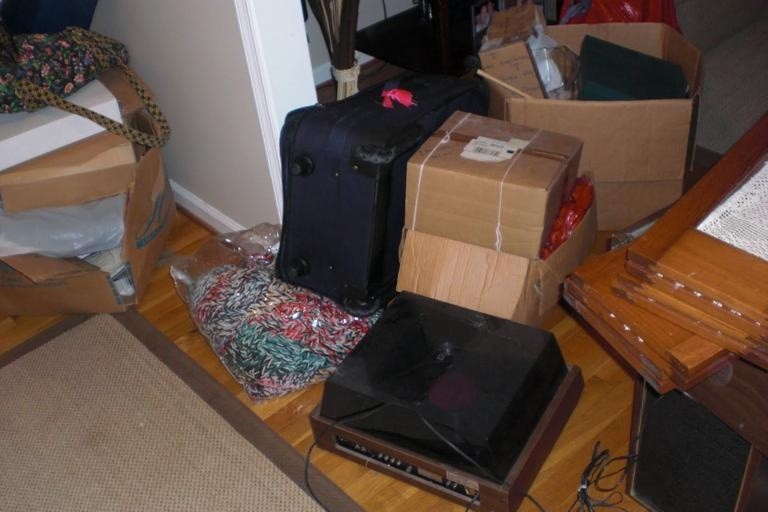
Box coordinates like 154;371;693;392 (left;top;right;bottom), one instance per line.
0;303;370;512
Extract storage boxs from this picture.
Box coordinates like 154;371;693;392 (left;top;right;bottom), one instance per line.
477;26;699;234
3;81;174;314
398;107;597;327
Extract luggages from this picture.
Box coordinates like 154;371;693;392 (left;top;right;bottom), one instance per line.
274;71;481;317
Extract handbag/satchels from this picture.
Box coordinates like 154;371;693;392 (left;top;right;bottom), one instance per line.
0;26;130;112
